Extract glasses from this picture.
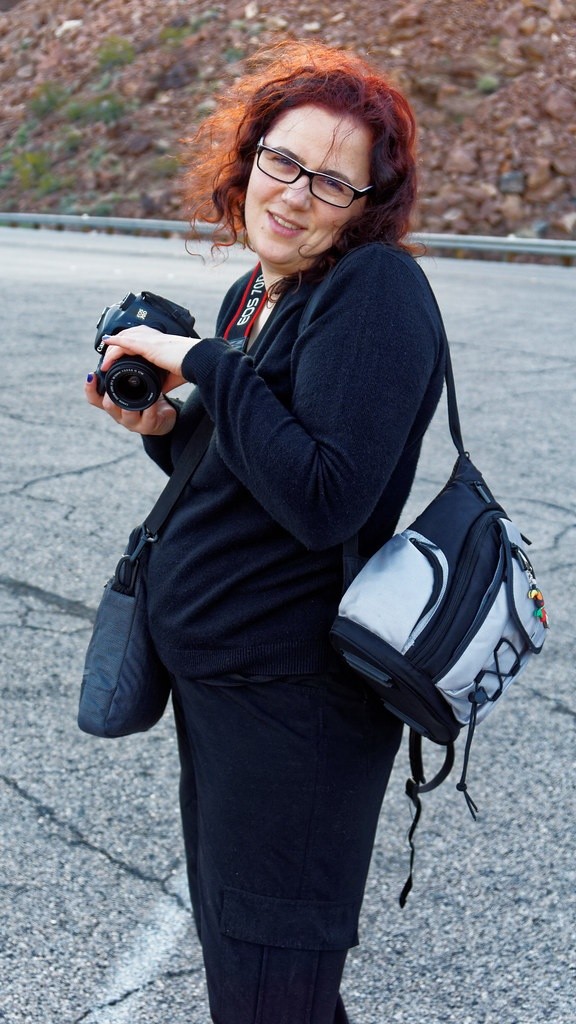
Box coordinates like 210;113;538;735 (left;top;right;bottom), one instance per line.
256;137;372;208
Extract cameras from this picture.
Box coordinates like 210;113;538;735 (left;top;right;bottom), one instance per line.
94;291;200;411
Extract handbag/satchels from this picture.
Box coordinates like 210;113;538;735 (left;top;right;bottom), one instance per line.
78;529;171;739
291;242;549;908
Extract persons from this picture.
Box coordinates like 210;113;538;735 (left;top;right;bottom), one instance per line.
85;43;446;1024
